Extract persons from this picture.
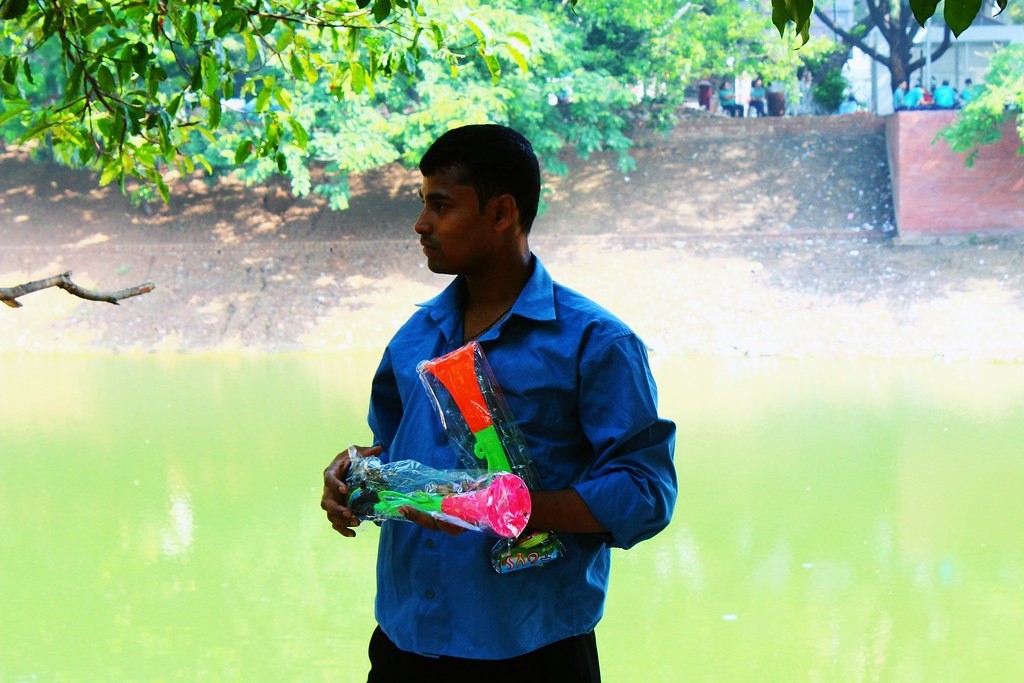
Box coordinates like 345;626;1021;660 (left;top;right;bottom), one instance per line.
320;124;678;683
749;78;773;117
841;96;857;114
893;78;974;110
697;79;744;119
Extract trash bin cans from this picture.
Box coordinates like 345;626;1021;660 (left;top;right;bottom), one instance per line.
697;80;711;111
767;91;785;116
